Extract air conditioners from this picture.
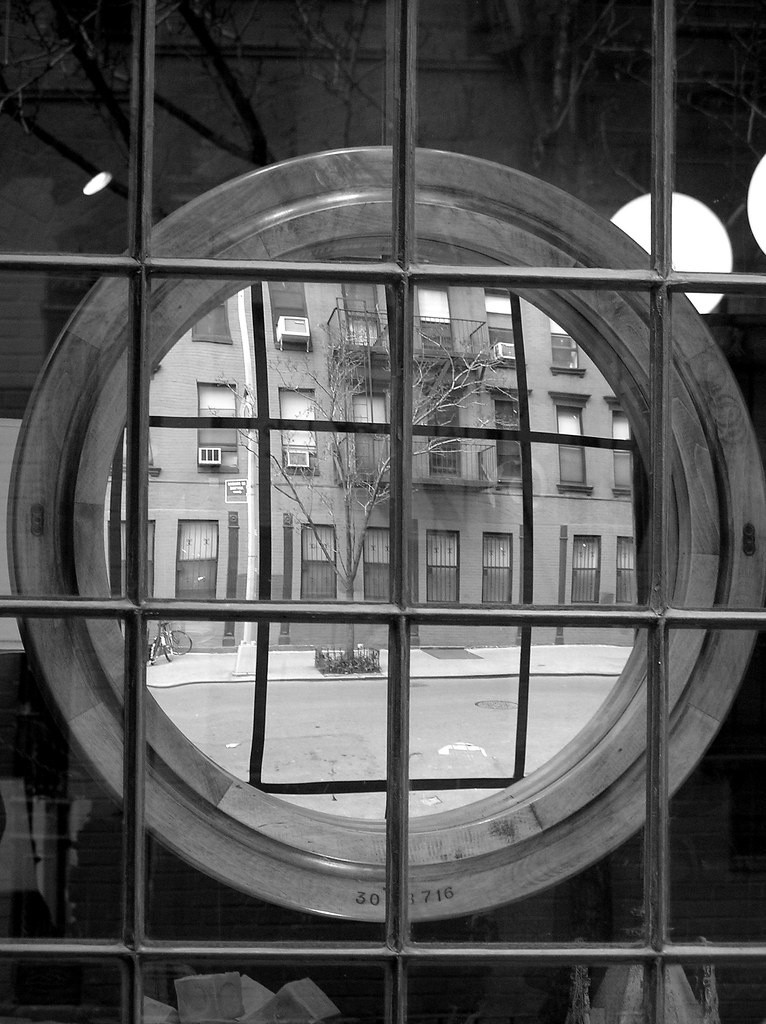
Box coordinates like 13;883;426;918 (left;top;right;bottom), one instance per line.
198;448;221;466
276;316;310;343
285;450;309;468
494;342;516;360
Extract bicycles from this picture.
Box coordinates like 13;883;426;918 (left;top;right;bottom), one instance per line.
148;618;192;665
